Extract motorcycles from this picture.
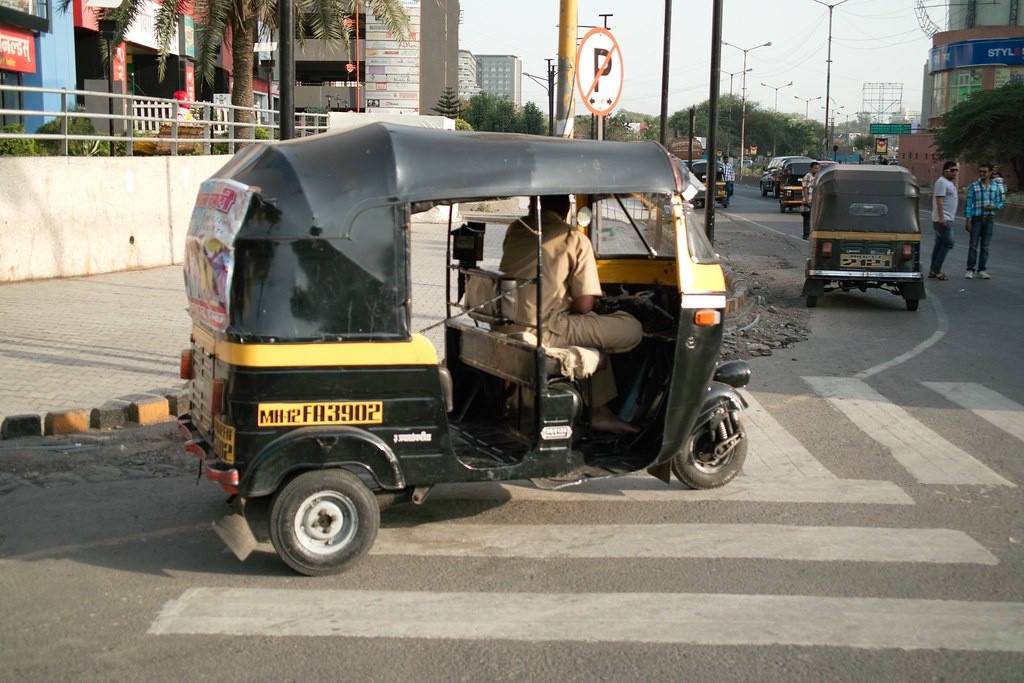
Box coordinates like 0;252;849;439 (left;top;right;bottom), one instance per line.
802;165;927;312
779;157;817;213
691;160;729;209
176;121;752;577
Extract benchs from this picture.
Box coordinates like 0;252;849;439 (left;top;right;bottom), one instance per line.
155;126;202;156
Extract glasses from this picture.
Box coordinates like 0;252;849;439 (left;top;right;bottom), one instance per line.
979;170;989;173
945;168;958;172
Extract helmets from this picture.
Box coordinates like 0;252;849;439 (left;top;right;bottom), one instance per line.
800;204;811;217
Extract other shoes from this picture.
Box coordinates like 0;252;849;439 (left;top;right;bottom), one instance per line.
803;235;809;239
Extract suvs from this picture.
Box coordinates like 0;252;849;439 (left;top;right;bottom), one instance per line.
759;155;798;198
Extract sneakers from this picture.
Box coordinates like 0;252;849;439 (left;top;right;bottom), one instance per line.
965;269;974;279
976;271;990;279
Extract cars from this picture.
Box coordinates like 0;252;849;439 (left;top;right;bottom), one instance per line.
738;159;752;167
817;160;839;170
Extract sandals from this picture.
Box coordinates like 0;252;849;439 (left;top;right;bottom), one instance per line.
929;274;934;278
930;271;948;281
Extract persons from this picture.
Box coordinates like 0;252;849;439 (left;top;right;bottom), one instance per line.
990;165;1001;179
964;164;1003;280
801;162;820;239
498;195;643;434
928;161;959;282
719;156;735;208
166;89;200;126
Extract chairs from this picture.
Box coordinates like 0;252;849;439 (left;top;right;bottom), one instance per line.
447;268;607;388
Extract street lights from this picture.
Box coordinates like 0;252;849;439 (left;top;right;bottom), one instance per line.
720;40;773;175
720;68;753;157
761;81;794;157
837;111;859;160
819;106;845;122
793;95;822;157
